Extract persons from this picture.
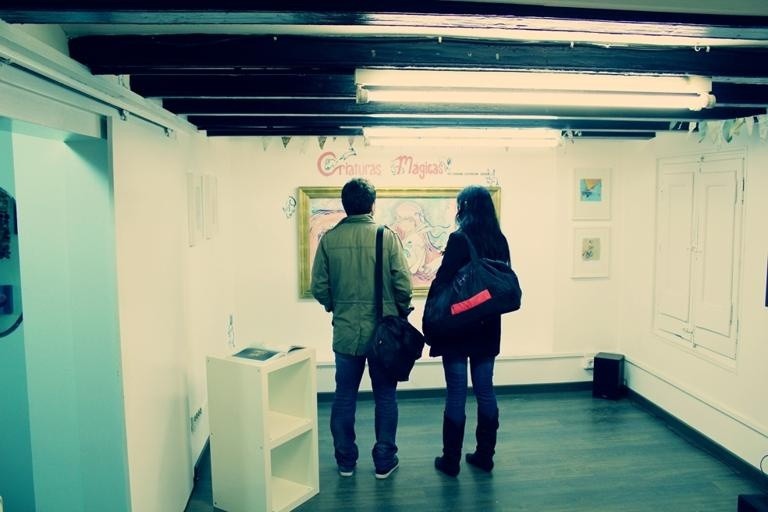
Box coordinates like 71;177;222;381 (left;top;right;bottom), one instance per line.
420;184;514;475
387;199;446;289
311;176;413;479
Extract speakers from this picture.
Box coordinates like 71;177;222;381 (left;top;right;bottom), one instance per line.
592;353;625;400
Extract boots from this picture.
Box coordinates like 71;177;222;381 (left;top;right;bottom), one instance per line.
466;409;499;471
435;410;466;477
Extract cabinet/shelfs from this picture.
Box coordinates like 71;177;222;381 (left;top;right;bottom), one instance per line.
205;341;320;512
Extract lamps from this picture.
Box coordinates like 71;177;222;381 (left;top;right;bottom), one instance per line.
348;70;718;110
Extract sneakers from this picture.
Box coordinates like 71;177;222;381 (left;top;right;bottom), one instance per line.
375;454;399;479
339;461;357;477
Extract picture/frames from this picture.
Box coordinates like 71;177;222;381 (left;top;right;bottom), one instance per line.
296;185;502;301
567;163;614;279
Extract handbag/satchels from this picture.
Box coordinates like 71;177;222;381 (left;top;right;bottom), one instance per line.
370;314;426;383
424;254;520;336
